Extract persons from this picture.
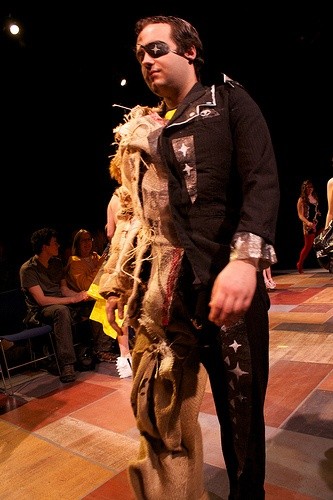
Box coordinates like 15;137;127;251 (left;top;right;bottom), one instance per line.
20;228;119;382
296;180;325;274
65;229;134;378
100;16;278;500
325;177;333;230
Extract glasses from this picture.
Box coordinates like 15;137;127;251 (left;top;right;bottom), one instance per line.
80;238;95;241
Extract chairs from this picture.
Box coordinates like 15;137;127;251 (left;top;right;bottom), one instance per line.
0;288;61;397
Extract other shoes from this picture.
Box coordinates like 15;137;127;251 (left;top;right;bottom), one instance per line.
61;365;74;382
265;279;274;289
269;278;277;286
95;351;117;362
